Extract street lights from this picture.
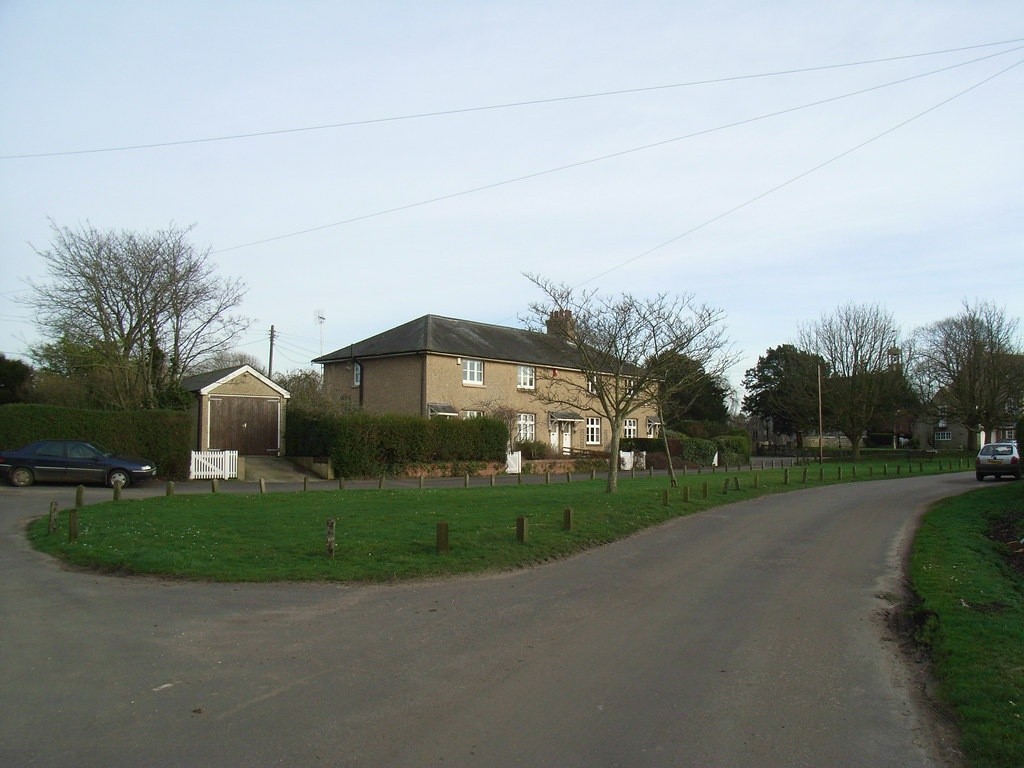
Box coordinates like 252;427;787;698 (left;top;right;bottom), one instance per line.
893;410;903;449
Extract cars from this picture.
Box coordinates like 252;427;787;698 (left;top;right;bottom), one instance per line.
976;443;1020;481
993;439;1019;455
0;440;157;489
